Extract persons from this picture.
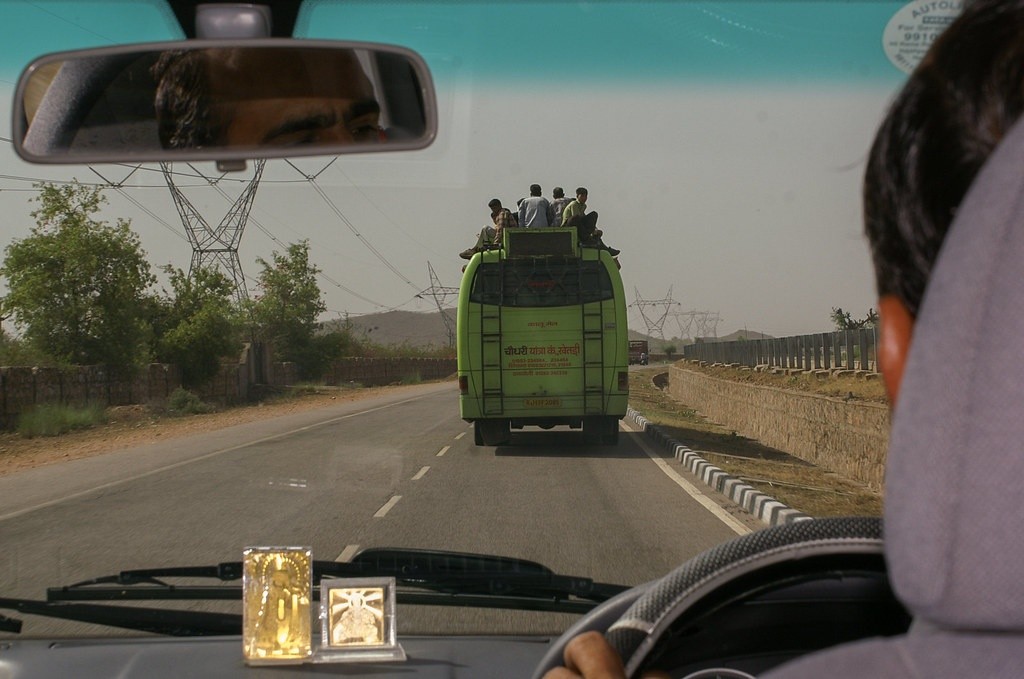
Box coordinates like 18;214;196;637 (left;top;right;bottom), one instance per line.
460;184;620;274
155;46;382;151
542;0;1024;679
641;352;648;363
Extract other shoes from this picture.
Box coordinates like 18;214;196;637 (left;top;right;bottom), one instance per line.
459;248;476;259
608;247;621;256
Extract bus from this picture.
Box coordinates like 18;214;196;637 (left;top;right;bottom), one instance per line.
627;340;652;365
458;227;627;448
